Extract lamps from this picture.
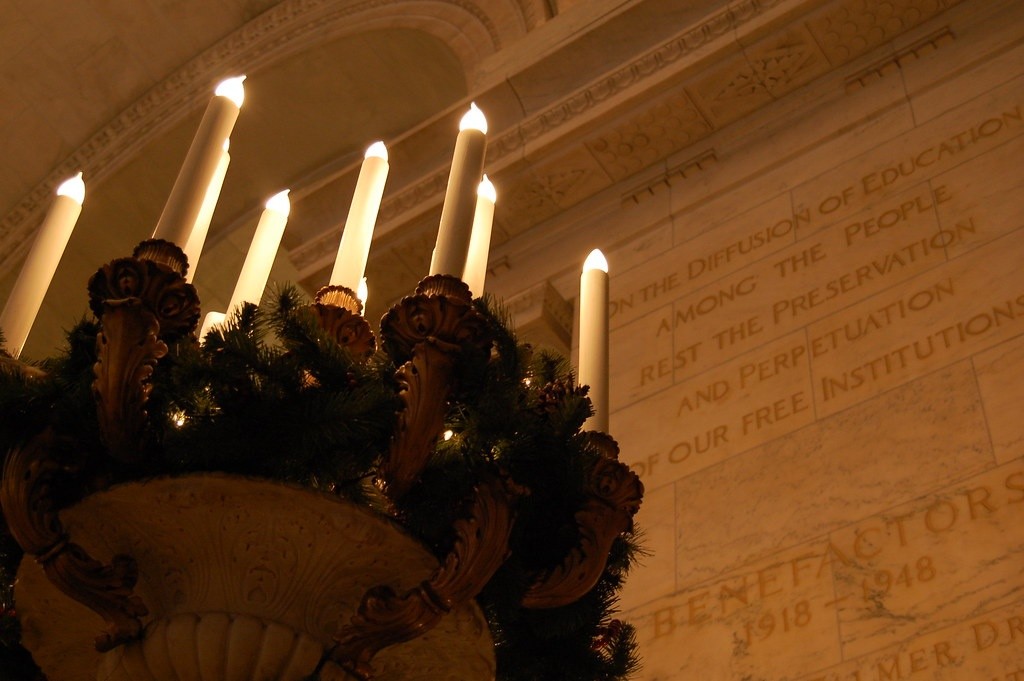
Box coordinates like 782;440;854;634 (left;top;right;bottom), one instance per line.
0;70;652;681
